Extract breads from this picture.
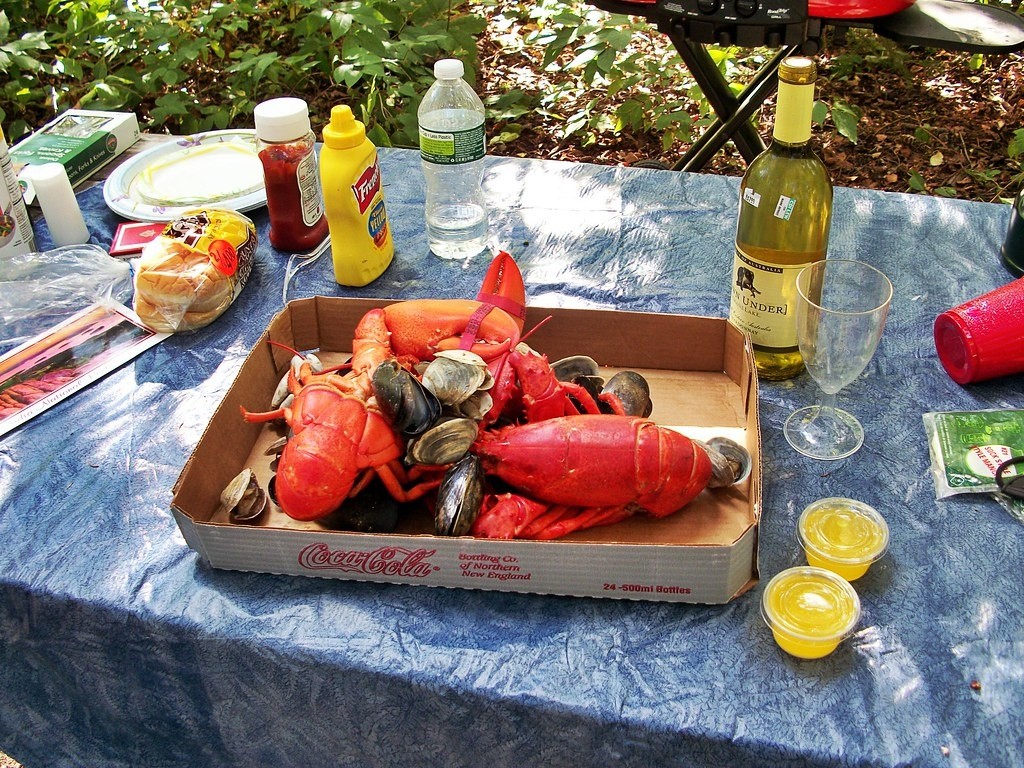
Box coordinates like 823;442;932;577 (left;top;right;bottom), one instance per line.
132;208;257;332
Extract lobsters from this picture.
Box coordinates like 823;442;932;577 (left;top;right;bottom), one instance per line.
240;249;713;539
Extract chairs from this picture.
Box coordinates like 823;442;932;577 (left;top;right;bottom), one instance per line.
584;0;917;174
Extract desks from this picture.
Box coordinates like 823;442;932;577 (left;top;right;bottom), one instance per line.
0;132;1024;768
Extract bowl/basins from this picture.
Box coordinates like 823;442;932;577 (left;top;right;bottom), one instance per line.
760;566;863;659
797;498;890;581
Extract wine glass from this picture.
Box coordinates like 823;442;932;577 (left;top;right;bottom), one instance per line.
782;258;894;461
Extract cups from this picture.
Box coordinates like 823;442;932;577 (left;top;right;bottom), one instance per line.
935;275;1024;384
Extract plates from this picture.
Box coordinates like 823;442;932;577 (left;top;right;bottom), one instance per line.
101;129;268;222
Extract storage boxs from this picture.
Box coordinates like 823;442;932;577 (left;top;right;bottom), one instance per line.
168;296;764;606
7;108;142;206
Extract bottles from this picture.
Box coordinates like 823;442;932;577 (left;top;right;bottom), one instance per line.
255;98;328;252
729;57;834;381
416;59;489;259
318;104;395;287
